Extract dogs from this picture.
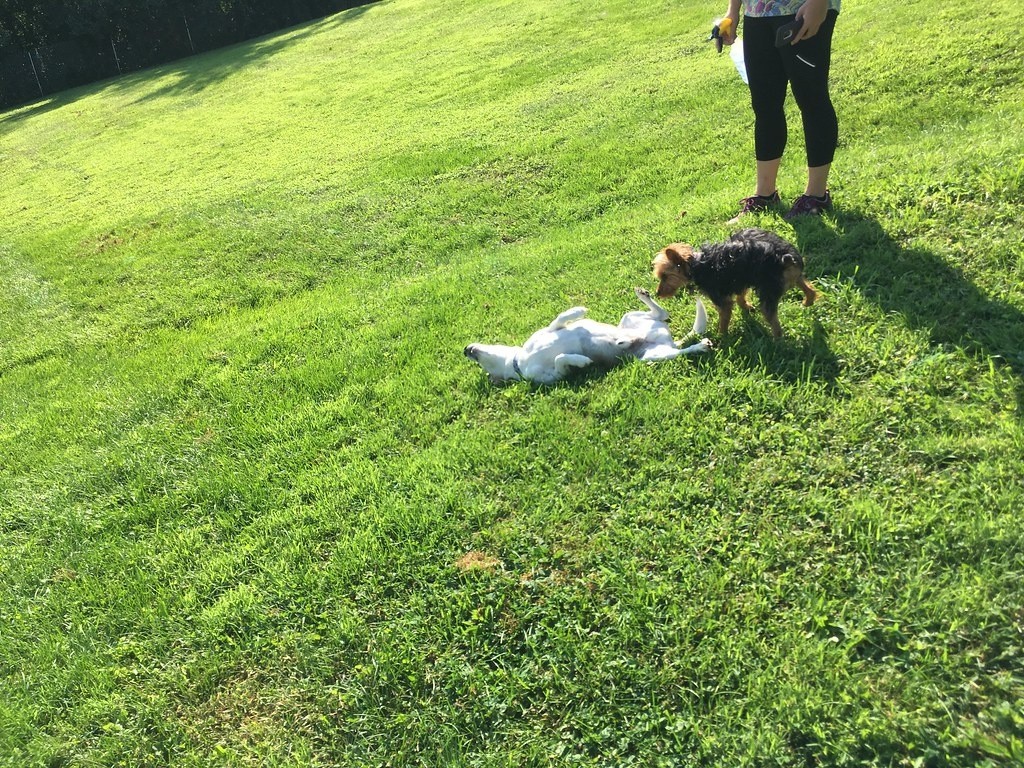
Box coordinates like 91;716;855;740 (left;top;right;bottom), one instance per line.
650;226;817;342
462;287;713;385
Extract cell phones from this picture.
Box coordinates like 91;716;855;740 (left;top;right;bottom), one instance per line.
775;17;805;47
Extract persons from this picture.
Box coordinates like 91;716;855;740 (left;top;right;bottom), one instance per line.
713;1;840;226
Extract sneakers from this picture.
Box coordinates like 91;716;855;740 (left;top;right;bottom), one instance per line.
724;190;781;224
783;190;833;219
